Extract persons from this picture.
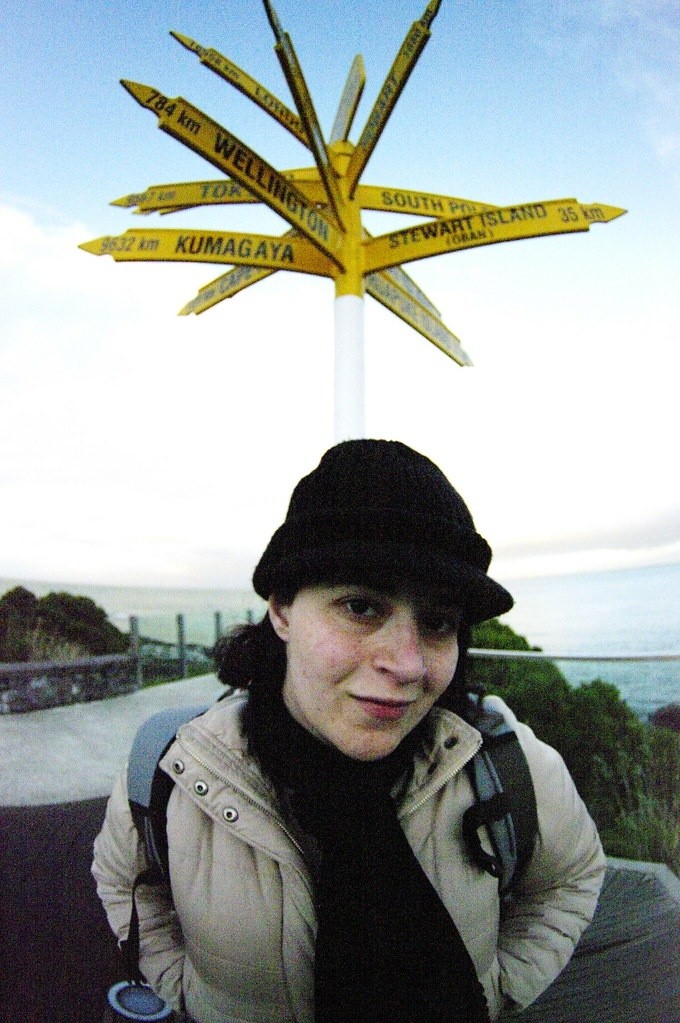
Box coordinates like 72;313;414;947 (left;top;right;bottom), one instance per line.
91;441;606;1023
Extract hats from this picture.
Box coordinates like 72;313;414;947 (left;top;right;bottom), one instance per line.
253;436;513;624
